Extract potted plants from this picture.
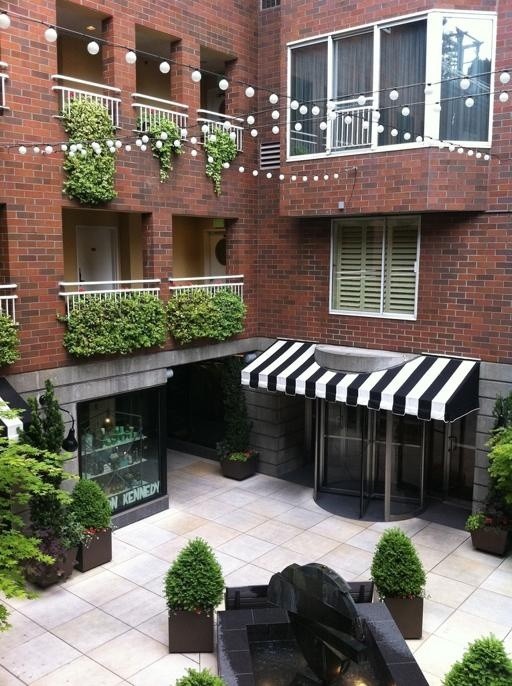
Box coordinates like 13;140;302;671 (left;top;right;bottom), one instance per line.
68;477;115;572
371;524;427;640
468;394;512;556
162;535;227;654
216;381;260;481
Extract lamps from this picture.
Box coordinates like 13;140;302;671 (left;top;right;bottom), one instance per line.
42;396;81;454
